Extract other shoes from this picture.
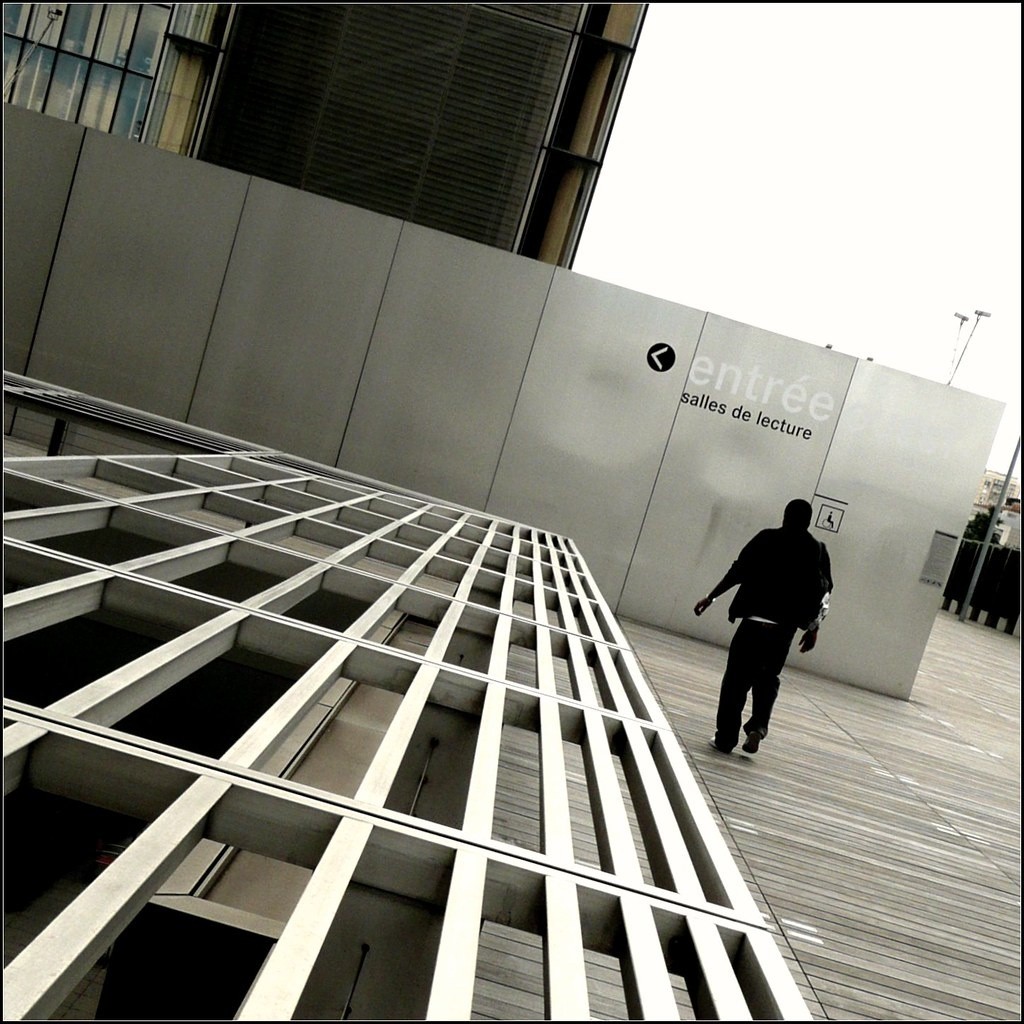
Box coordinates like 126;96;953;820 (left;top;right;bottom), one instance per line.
742;731;760;753
710;736;716;747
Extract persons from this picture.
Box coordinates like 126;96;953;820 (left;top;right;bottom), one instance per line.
693;498;834;755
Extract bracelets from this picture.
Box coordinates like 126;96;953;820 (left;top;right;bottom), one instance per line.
705;594;716;602
